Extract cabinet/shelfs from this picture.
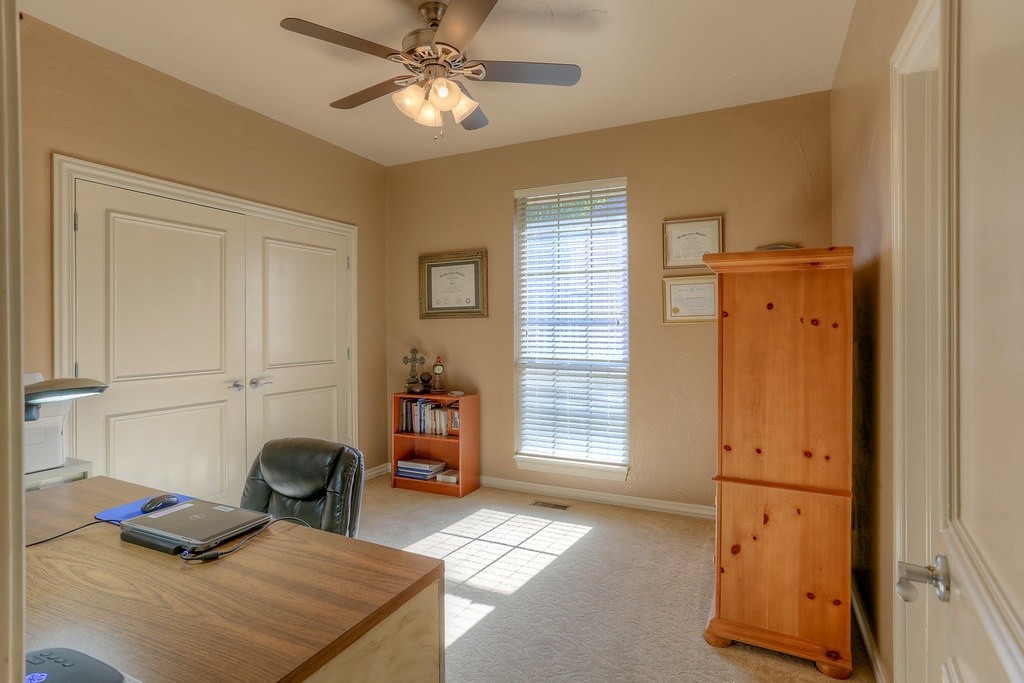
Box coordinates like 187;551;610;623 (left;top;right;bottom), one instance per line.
26;475;447;683
703;244;859;680
391;390;481;498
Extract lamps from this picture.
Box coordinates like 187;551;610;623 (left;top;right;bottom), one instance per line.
391;77;479;127
26;378;126;683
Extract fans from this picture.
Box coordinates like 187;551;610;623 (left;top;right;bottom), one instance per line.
280;0;582;129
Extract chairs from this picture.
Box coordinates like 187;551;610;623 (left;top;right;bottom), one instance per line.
240;438;364;540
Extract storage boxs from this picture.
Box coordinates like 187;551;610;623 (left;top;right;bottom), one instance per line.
436;469;459;483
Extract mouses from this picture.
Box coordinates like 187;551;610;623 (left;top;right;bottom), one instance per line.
141;494;179;514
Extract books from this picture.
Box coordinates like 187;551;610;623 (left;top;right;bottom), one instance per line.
437;470;459;482
396;458;446;480
400;399;448;436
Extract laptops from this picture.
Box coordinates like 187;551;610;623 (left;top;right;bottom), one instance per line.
121;499;272;553
